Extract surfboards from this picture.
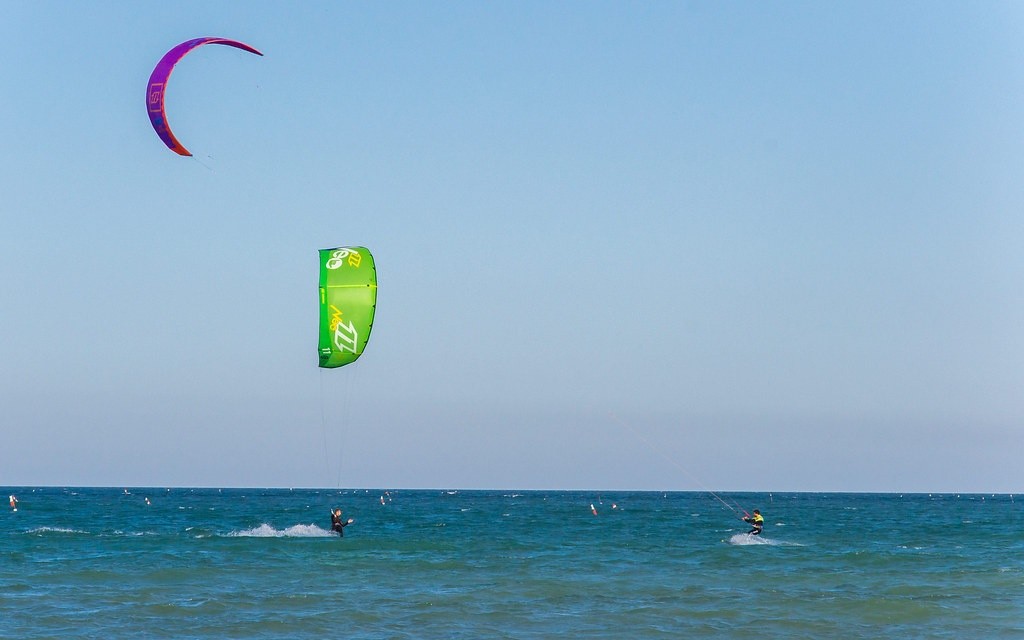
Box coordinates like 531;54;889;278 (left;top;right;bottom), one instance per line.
721;539;734;544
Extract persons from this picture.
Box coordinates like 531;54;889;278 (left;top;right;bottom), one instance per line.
742;510;764;536
331;509;354;536
